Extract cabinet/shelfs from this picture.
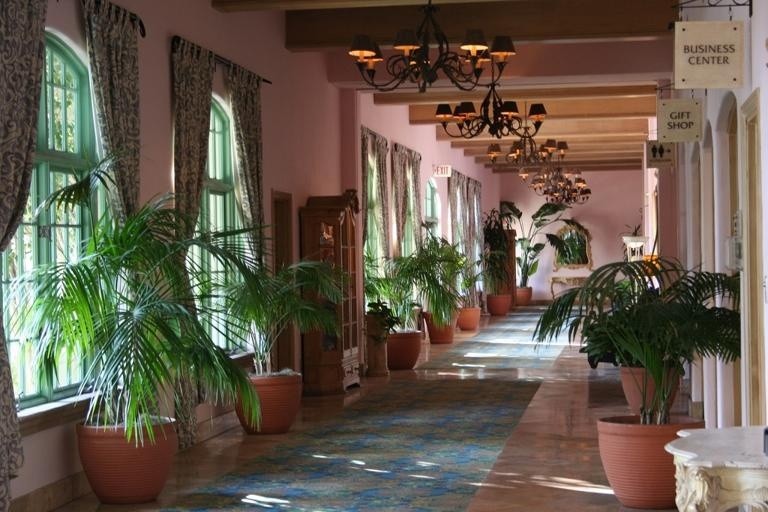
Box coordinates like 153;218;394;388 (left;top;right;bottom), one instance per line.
299;212;360;365
304;359;362;394
504;230;516;311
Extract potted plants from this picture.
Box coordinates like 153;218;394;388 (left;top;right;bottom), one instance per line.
500;201;573;306
416;232;482;345
3;147;272;503
210;260;354;436
362;233;424;377
476;209;513;316
532;257;742;509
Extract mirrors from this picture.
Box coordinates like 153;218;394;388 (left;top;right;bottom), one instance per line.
552;219;596;273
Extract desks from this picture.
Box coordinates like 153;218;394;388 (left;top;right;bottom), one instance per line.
663;425;768;512
550;277;593;312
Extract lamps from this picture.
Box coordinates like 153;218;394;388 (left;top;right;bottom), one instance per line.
435;56;548;140
486;102;592;209
347;0;517;94
